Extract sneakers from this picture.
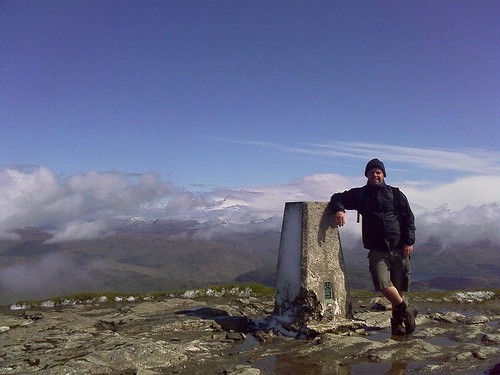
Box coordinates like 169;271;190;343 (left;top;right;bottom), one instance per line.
403;304;418;334
390;318;407;336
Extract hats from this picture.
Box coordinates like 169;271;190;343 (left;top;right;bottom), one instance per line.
364;158;386;178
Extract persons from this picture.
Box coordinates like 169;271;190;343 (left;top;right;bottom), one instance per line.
331;159;418;336
